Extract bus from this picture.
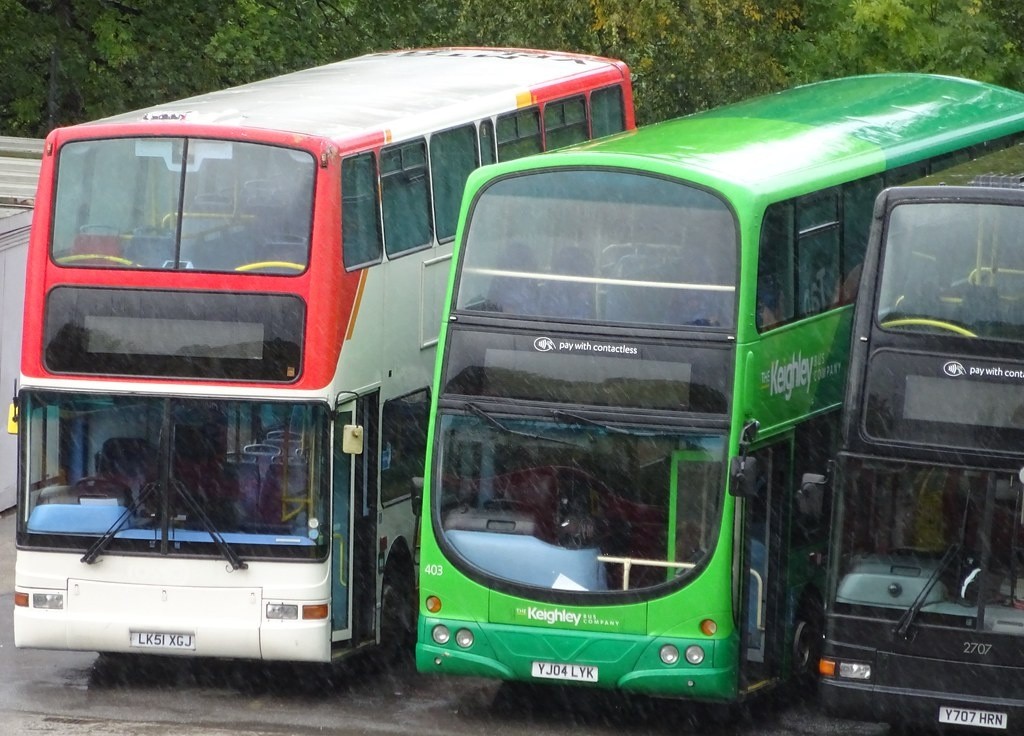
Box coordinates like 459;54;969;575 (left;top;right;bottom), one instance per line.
10;45;635;676
415;71;1024;727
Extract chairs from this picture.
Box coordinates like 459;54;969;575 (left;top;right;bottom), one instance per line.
94;429;310;534
72;170;313;271
